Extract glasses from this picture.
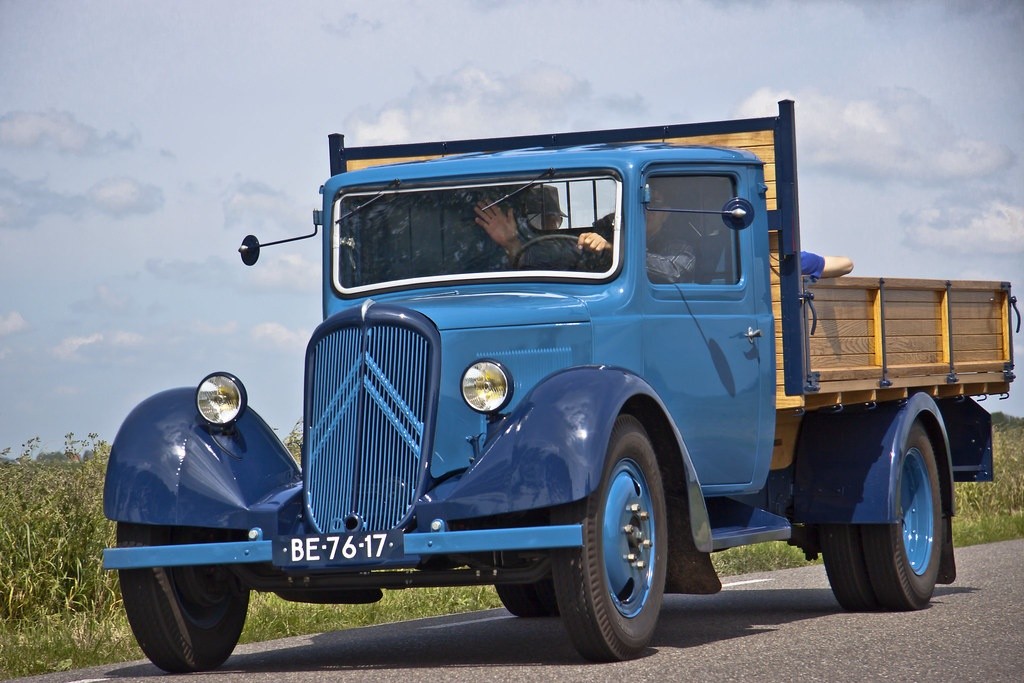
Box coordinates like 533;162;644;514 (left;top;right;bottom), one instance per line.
651;197;662;207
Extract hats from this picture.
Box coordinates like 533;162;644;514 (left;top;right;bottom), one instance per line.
526;186;568;217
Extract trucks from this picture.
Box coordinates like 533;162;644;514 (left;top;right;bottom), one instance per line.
101;97;1021;676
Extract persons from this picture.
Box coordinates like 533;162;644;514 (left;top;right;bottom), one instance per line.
471;174;699;286
796;251;857;279
520;186;571;230
